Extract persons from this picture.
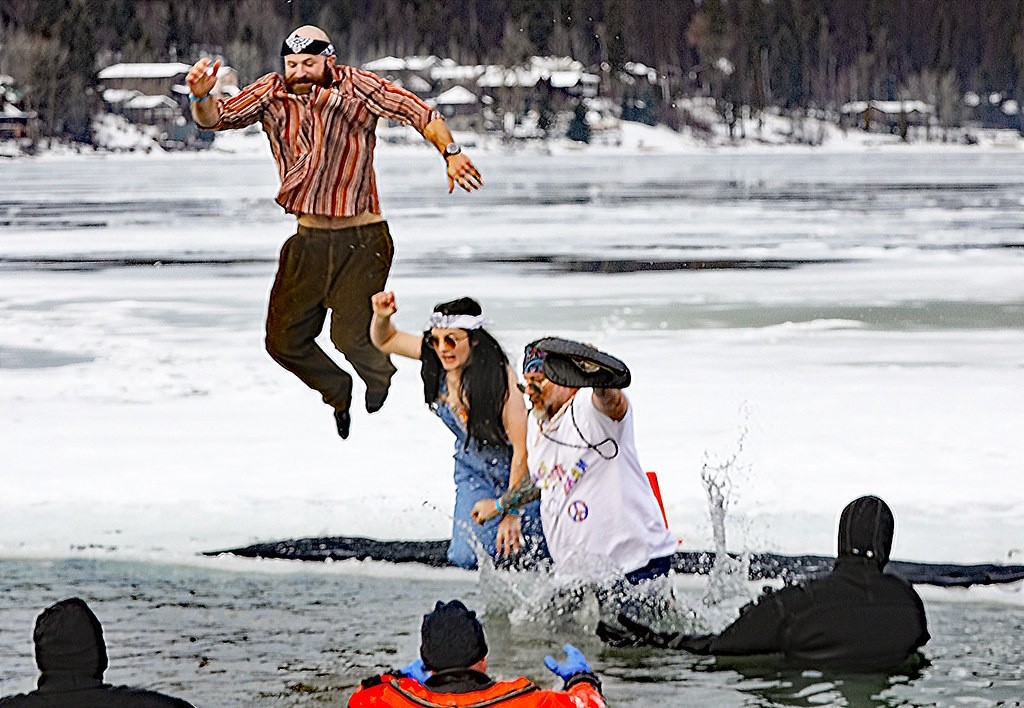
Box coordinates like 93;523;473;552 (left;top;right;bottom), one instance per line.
369;290;542;564
0;598;197;708
471;337;676;630
597;497;929;661
187;25;485;439
347;600;609;708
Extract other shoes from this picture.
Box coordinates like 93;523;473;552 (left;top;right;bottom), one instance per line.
365;388;388;413
334;404;351;439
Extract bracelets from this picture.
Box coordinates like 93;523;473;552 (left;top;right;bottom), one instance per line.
496;499;504;512
506;509;520;514
189;94;209;101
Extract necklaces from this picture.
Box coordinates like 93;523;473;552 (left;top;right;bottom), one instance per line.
533;396;618;459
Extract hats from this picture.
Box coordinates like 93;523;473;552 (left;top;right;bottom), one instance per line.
523;337;554;373
34;597;109;679
420;599;488;672
837;495;894;570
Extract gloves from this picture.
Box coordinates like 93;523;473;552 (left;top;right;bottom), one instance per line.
544;643;593;679
594;613;682;650
400;659;430;686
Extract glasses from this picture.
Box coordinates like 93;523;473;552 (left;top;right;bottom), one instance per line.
516;379;550;395
425;333;468;351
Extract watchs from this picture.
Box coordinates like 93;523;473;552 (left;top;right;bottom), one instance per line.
443;143;461;159
